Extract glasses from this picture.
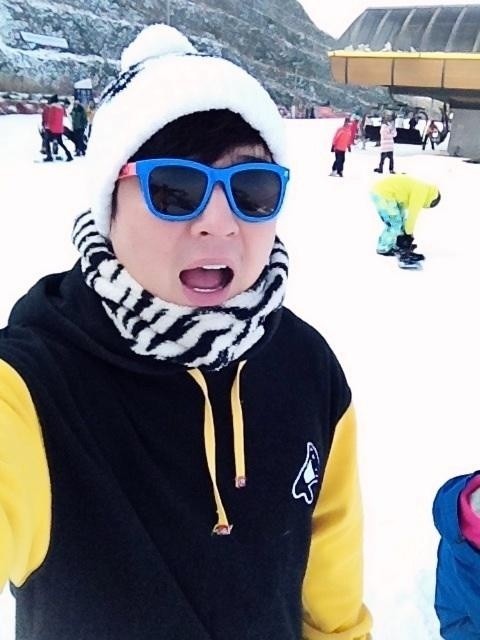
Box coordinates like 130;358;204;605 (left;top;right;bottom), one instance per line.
116;159;291;222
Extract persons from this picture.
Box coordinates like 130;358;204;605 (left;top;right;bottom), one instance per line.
43;94;74;162
367;173;441;270
86;98;95;138
374;117;398;174
409;118;418;129
431;469;480;639
38;98;57;156
422;120;440;150
0;23;373;640
70;98;85;157
327;122;354;177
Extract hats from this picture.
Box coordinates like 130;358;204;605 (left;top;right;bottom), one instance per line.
73;21;291;238
